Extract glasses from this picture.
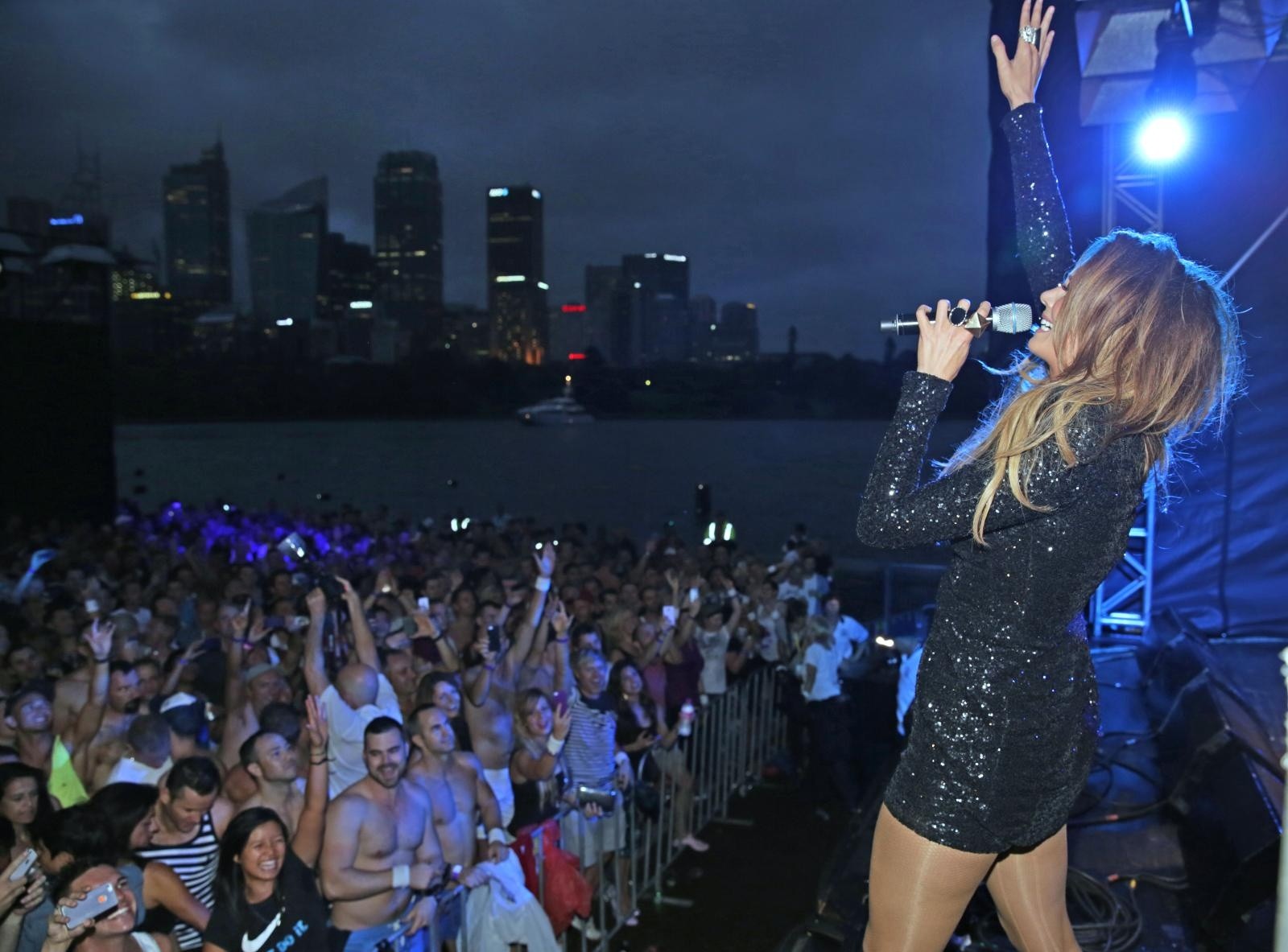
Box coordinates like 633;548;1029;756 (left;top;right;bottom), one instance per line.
16;699;55;715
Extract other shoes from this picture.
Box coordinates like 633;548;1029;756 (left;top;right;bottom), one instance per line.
604;887;640;928
673;833;710;851
571;915;601;942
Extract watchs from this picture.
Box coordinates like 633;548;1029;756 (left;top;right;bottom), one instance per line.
451;866;462;882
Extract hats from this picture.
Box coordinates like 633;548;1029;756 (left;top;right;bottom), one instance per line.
148;692;207;728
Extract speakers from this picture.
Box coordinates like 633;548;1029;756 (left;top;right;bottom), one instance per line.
1139;606;1284;952
1075;0;1288;128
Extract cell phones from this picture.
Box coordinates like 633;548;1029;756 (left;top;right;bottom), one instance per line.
487;625;501;652
552;690;567;718
689;588;699;602
10;848;38;882
61;882;119;930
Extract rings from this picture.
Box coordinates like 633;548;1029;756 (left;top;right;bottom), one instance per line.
963;312;990;337
949;307;967;327
1018;24;1037;45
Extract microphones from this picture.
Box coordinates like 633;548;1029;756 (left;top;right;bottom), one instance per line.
881;302;1032;336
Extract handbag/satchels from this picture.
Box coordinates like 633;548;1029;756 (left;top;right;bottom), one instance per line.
772;665;791;708
540;818;594;935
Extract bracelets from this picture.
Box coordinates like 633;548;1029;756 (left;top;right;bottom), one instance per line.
687;614;696;620
232;638;243;643
176;657;190;665
481;663;493;673
555;636;569;645
656;637;663;643
308;750;327;755
245;639;257;647
372;591;378;598
93;655;109;664
308;757;337;765
645;550;651;558
396;590;404;599
505;601;513;610
434;631;444;642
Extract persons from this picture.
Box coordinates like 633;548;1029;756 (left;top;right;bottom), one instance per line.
0;498;876;952
854;0;1249;952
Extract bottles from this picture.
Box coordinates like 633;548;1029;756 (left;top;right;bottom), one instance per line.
679;698;693;736
